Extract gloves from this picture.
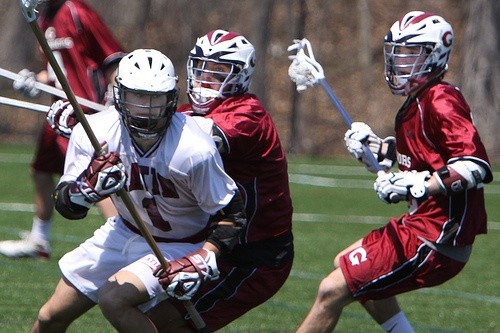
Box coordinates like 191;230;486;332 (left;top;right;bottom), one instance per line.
373;169;430;204
12;68;41;98
46;98;76;138
343;121;397;173
70;141;128;206
153;247;221;298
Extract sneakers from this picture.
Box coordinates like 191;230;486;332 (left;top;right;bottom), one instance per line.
0;226;51;259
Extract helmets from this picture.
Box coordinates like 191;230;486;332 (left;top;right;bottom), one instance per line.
113;48;180;137
186;28;256;115
384;10;453;97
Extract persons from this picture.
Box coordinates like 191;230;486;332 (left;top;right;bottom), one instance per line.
295;11;494;333
29;49;249;333
145;28;295;333
0;0;127;259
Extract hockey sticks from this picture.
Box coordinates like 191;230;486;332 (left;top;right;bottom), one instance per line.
18;0;206;329
0;96;51;112
288;37;400;204
0;67;107;112
